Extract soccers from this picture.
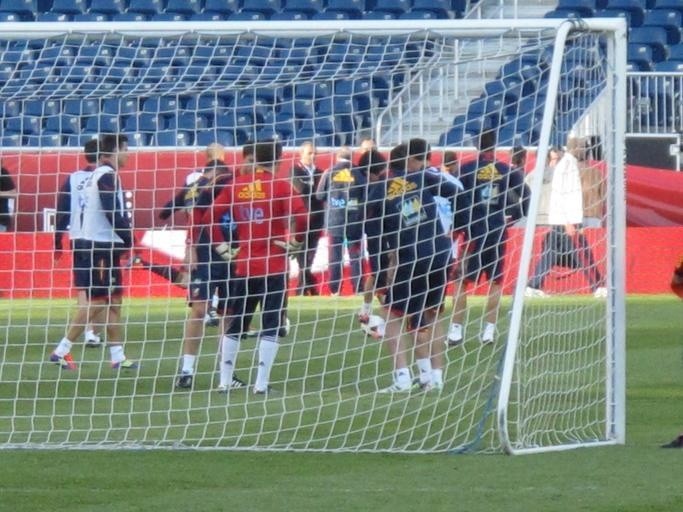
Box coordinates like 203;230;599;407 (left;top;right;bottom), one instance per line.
360;315;385;339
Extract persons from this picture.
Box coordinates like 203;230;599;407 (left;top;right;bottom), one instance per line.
48;135;139;371
54;138;103;348
660;263;682;448
124;122;608;394
0;166;17;233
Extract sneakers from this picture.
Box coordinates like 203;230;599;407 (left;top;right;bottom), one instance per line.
48;351;77;369
112;359;142;369
204;306;290;337
447;333;463;345
525;286;544;297
481;335;494;344
378;373;443;394
219;378;247;392
595;288;607;296
85;331;102;346
175;372;192;388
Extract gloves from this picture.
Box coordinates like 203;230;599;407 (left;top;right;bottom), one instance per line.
216;243;239;260
274;237;304;259
